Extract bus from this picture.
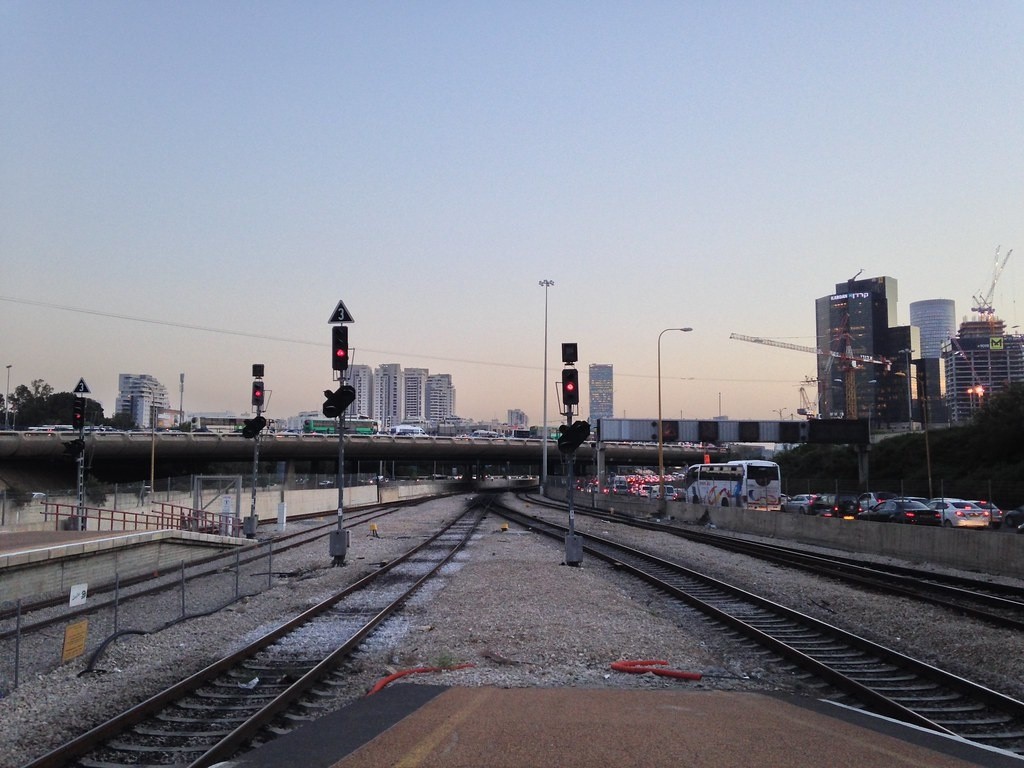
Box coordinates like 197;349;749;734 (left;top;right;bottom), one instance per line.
683;458;782;512
189;415;275;438
302;417;379;438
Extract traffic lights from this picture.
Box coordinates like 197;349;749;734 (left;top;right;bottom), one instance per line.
252;364;265;377
252;381;265;406
557;420;591;454
242;415;267;439
332;326;348;370
562;369;579;406
322;385;356;418
73;397;86;430
561;342;578;362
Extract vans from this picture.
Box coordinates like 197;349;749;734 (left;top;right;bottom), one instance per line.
390;424;431;440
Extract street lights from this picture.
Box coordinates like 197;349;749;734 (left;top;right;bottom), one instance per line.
4;364;12;431
141;381;155;493
899;348;917;431
539;279;555;494
658;327;693;500
894;371;932;497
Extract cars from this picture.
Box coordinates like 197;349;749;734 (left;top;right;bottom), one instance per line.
358;473;540;485
319;480;334;487
26;491;46;499
574;468;687;502
781;491;1024;534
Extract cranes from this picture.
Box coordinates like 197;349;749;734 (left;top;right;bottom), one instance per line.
729;311;892;420
972;245;1015;335
